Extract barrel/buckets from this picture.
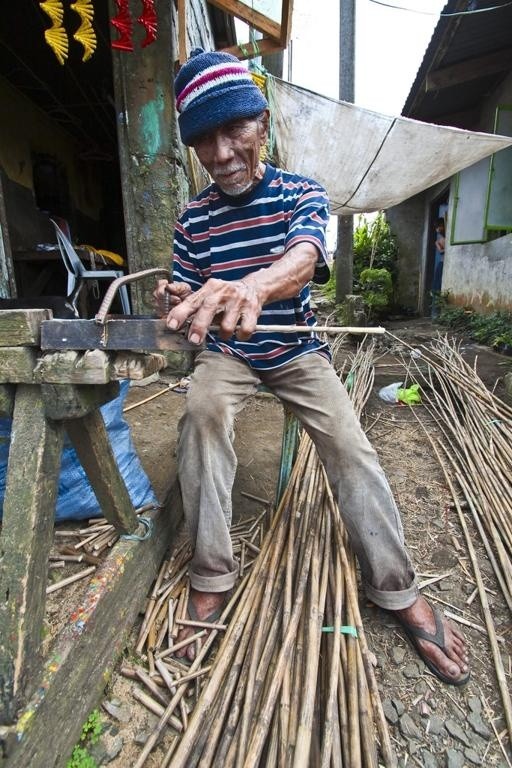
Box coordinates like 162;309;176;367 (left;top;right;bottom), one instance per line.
167;593;225;666
400;598;471;685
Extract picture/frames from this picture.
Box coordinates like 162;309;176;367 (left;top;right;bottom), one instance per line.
14;250;128;318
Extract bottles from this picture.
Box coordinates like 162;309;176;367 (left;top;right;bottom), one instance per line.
175;48;270;147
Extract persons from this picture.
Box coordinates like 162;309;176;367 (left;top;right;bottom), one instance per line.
435;218;446;252
153;49;470;686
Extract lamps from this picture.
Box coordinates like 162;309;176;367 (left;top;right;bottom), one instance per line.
51;219;131;317
